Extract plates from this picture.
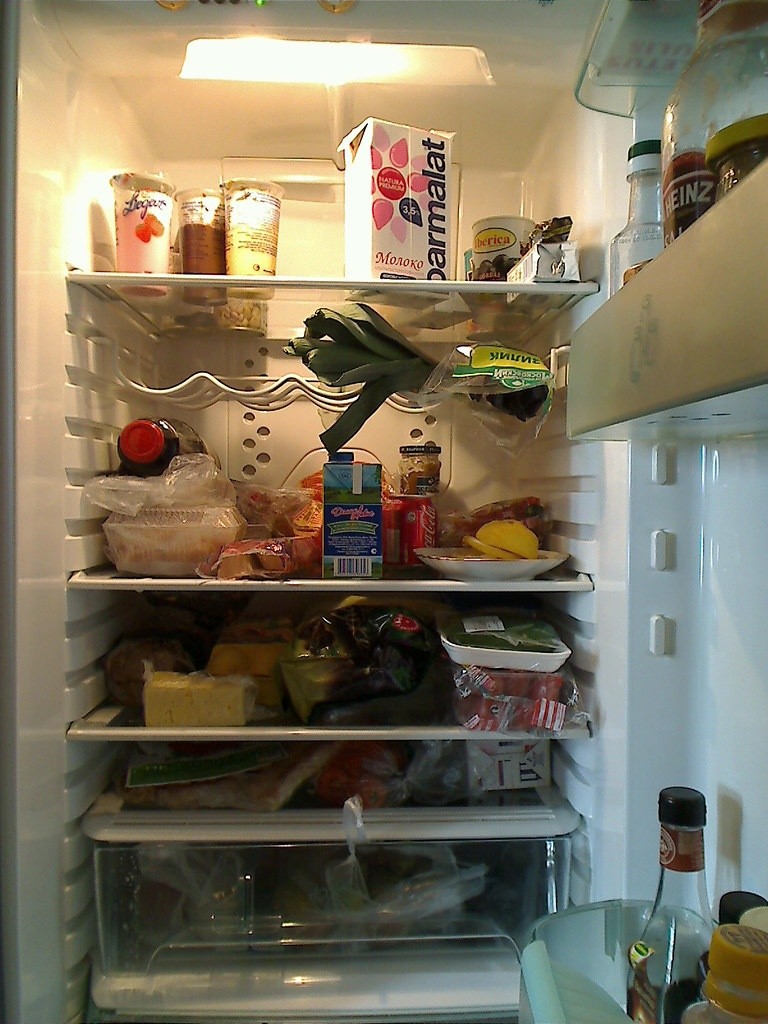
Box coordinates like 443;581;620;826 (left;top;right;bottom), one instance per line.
413;548;569;580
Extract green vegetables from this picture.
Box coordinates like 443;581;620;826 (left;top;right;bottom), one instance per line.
287;294;550;455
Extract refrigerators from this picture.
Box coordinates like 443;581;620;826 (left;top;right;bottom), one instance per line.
0;0;768;1024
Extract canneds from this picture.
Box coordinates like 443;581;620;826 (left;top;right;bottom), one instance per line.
400;446;442;495
384;495;438;569
704;112;768;207
471;214;535;280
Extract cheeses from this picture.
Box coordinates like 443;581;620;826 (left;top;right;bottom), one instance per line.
144;671;252;726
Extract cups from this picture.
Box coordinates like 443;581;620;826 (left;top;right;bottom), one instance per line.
175;188;226;306
221;178;283;299
110;173;174;297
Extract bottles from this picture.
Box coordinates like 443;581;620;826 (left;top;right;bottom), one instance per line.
627;787;714;1024
693;892;768;1002
608;141;663;298
680;924;768;1024
661;1;768;249
117;417;222;478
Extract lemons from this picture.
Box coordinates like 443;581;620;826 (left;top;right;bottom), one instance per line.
463;520;539;560
